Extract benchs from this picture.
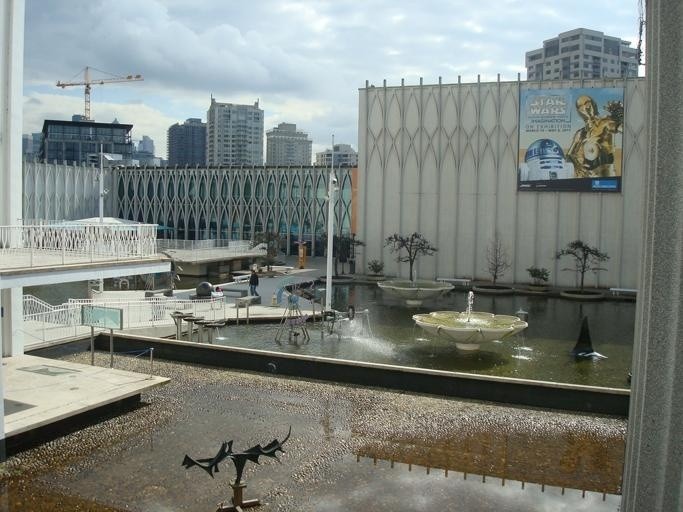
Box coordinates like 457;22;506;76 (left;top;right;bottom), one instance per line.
218;287;263;307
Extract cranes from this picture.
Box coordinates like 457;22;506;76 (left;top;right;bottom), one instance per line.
55;66;144;121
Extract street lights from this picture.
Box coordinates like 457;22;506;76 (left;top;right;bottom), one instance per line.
98;139;104;226
326;133;337;311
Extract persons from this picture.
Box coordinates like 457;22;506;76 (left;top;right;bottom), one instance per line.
248;269;259;295
